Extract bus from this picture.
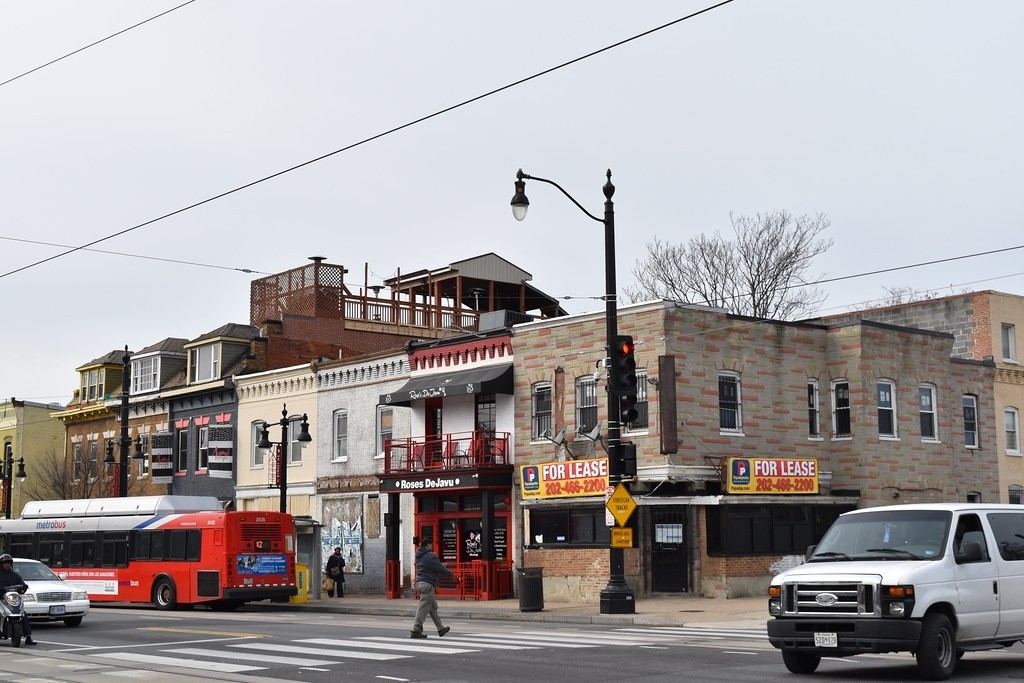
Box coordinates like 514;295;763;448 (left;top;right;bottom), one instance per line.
0;491;299;612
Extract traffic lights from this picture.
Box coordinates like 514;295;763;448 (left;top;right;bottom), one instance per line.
619;391;638;425
611;335;637;396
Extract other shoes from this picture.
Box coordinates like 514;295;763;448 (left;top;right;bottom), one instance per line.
439;626;450;636
25;638;38;645
410;630;427;638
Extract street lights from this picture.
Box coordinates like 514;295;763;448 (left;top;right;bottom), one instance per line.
0;444;27;520
253;402;313;513
510;168;636;615
103;344;150;497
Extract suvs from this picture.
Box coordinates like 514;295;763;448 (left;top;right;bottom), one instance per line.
766;501;1024;681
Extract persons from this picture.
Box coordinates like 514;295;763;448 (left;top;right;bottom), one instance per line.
326;548;345;597
0;554;37;645
410;538;455;638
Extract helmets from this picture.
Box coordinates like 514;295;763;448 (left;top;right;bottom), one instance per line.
0;554;13;564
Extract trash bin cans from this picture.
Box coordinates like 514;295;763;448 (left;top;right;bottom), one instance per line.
516;567;545;612
289;563;307;603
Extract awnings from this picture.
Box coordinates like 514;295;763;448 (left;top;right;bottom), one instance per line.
379;364;514;407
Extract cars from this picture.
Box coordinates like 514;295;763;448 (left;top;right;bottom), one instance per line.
12;557;90;628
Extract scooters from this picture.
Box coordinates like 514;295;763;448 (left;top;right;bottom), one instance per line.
0;584;26;648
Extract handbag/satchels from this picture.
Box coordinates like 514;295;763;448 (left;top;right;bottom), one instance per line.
322;577;334;591
331;566;340;578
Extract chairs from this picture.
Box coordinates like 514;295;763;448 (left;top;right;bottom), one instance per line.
399;445;425;471
479;438;505;463
450;440;481;466
430;441;458;470
